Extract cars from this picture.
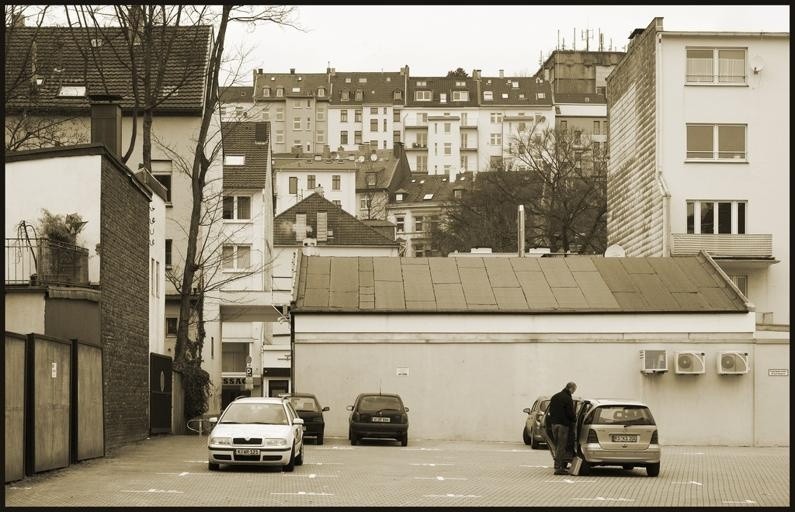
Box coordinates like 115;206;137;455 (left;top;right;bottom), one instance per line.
205;394;306;473
572;400;663;479
346;393;410;446
522;393;579;449
279;393;331;446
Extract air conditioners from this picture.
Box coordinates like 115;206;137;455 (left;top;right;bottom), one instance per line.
639;349;668;375
716;350;749;376
674;351;706;375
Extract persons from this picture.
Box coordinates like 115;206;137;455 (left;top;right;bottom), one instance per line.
549;381;577;475
